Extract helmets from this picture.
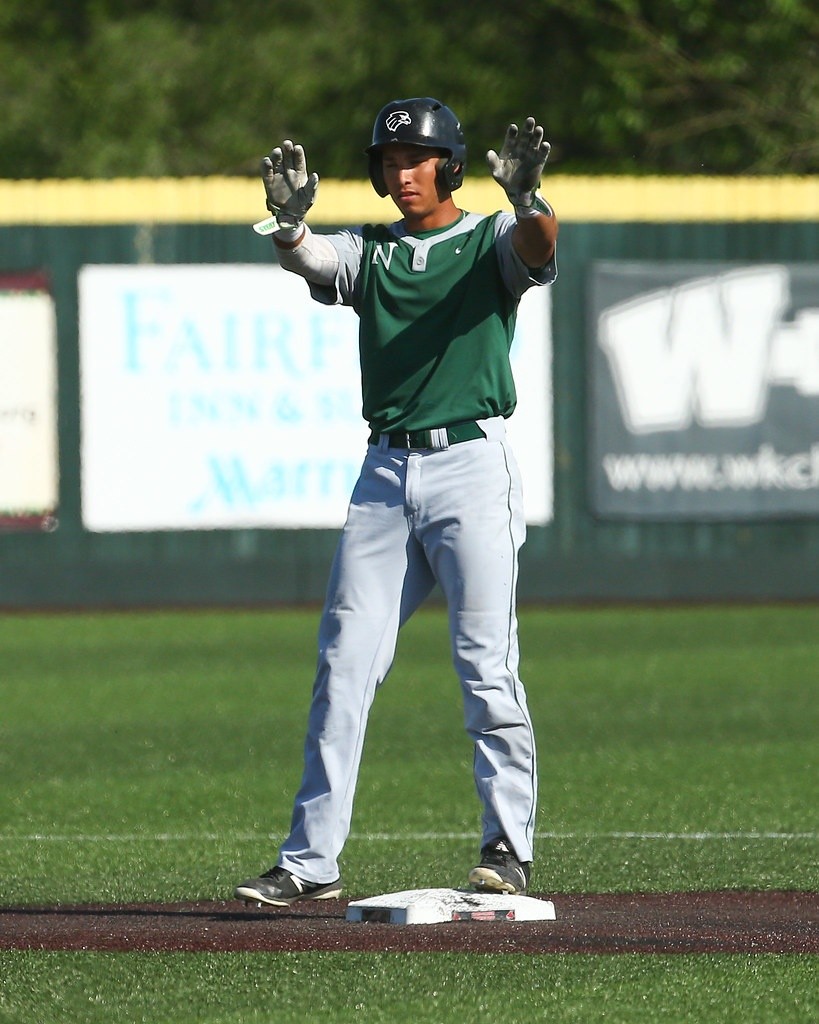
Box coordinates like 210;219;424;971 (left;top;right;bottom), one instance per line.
365;97;468;198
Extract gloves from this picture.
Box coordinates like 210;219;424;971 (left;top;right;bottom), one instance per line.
485;117;551;194
260;140;319;227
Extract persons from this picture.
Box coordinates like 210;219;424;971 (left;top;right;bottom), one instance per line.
234;94;554;907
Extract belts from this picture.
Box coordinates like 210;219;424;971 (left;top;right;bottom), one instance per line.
369;422;485;448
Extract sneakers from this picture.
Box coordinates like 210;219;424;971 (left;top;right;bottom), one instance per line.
469;839;529;896
235;866;343;907
